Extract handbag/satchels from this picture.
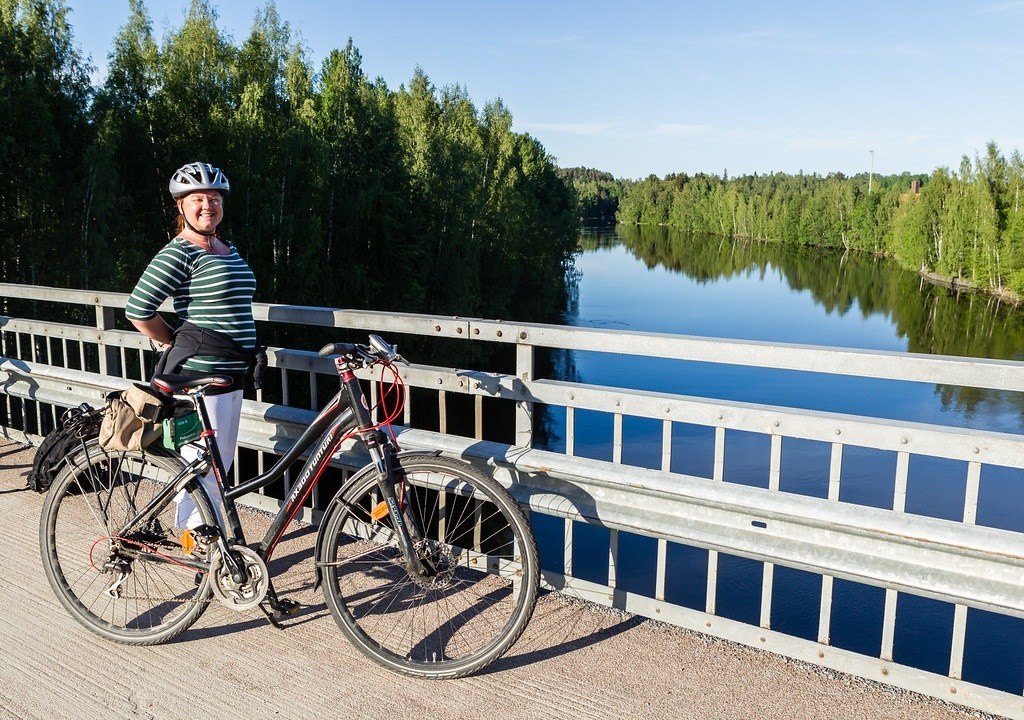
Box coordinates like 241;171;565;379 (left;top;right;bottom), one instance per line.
100;381;195;451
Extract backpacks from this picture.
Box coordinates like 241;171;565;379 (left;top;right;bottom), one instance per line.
26;423;99;496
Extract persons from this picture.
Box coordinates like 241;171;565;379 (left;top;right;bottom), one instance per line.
125;163;256;564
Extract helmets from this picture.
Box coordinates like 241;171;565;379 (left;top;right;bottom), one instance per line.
168;161;230;201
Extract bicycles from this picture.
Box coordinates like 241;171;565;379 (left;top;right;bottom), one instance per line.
37;332;542;682
